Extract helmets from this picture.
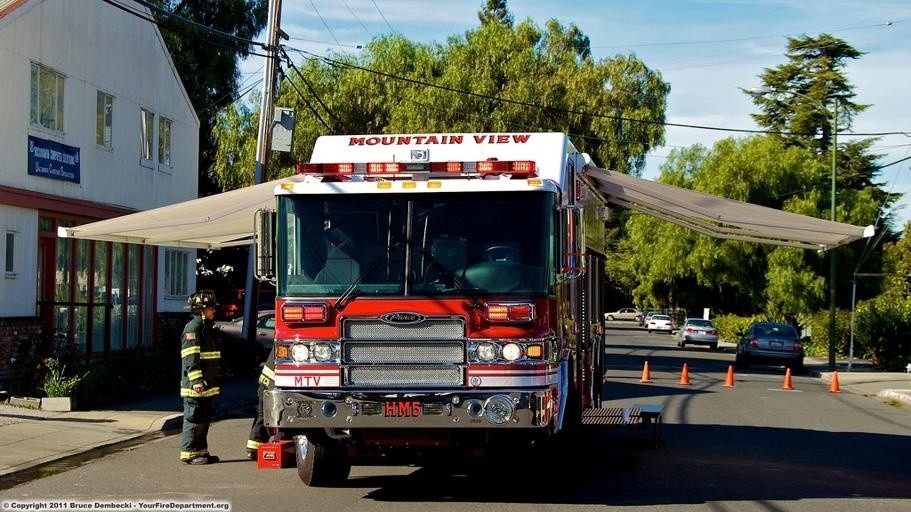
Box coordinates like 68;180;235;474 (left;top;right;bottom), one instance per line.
183;289;220;311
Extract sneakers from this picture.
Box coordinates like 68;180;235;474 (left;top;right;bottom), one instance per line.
181;453;220;464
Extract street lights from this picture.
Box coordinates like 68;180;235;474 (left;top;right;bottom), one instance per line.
740;86;838;371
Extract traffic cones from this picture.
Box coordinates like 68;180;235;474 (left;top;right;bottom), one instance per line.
640;360;652;383
828;371;840;393
781;368;792;390
722;365;735;388
678;362;691;386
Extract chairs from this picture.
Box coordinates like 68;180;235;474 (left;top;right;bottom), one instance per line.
424;240;467;284
312;238;365;285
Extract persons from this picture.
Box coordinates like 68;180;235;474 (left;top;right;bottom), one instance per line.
179;288;226;465
246;348;280;460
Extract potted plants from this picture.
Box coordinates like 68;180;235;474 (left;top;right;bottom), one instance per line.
37;357;91;411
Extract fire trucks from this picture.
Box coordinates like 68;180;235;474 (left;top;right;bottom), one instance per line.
253;131;608;487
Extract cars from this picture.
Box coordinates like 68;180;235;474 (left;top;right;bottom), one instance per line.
678;318;718;349
735;322;805;371
604;308;646;322
638;311;673;334
212;309;275;380
